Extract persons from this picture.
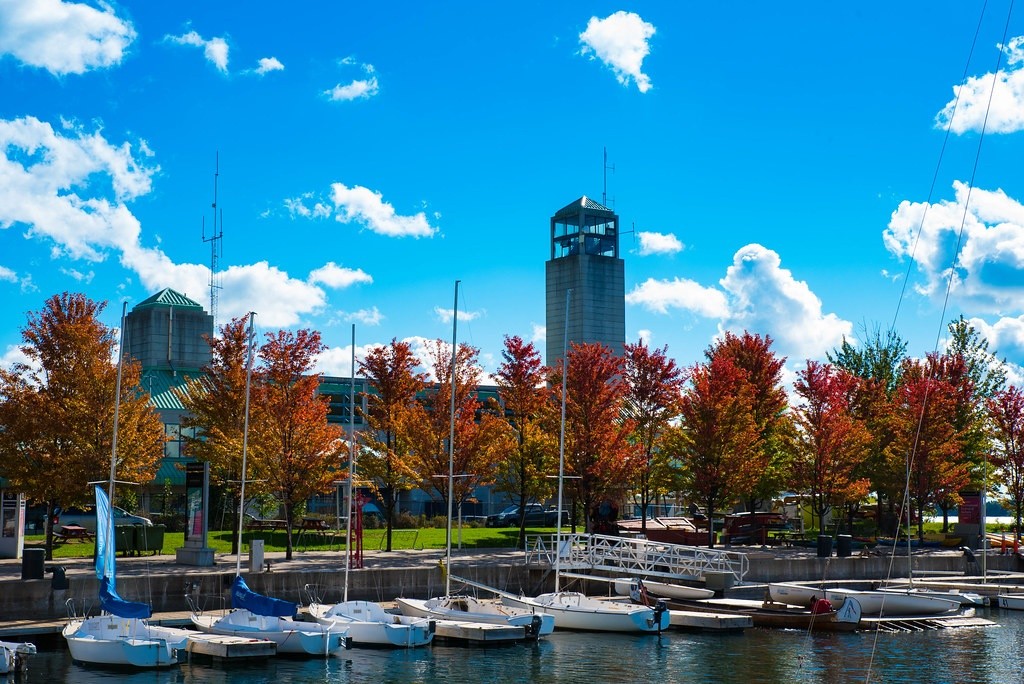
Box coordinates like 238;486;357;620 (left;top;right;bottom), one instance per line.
686;501;701;515
809;595;832;614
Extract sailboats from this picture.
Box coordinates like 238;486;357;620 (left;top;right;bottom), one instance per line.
394;281;554;639
192;312;360;656
498;290;671;632
62;302;191;669
307;324;436;647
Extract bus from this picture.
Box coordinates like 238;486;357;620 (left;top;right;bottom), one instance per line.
778;491;856;522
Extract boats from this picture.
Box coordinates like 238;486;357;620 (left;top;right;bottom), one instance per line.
877;574;1024;610
770;580;960;614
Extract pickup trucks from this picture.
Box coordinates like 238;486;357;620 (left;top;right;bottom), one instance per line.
485;503;569;528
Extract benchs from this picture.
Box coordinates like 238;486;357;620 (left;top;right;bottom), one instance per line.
247;525;288;529
52;532;65;538
766;539;816;542
86;533;96;537
293;523;330;529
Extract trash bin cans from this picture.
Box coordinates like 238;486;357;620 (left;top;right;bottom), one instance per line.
837;534;852;556
115;524;165;557
23;548;45;579
818;535;833;557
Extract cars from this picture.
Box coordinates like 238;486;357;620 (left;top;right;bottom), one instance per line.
42;504;151;534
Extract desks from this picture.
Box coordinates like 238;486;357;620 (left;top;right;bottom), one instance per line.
247;519;288;533
297;517;325;539
53;525;95;543
770;533;808;550
